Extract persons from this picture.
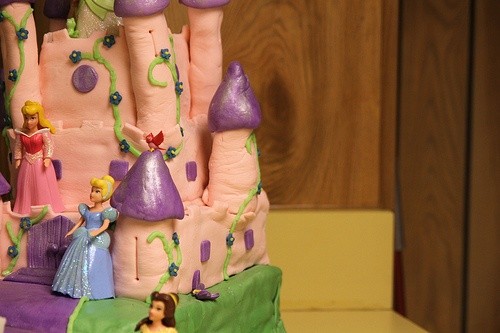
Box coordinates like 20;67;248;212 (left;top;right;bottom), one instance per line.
10;100;67;215
134;290;180;333
50;175;121;301
